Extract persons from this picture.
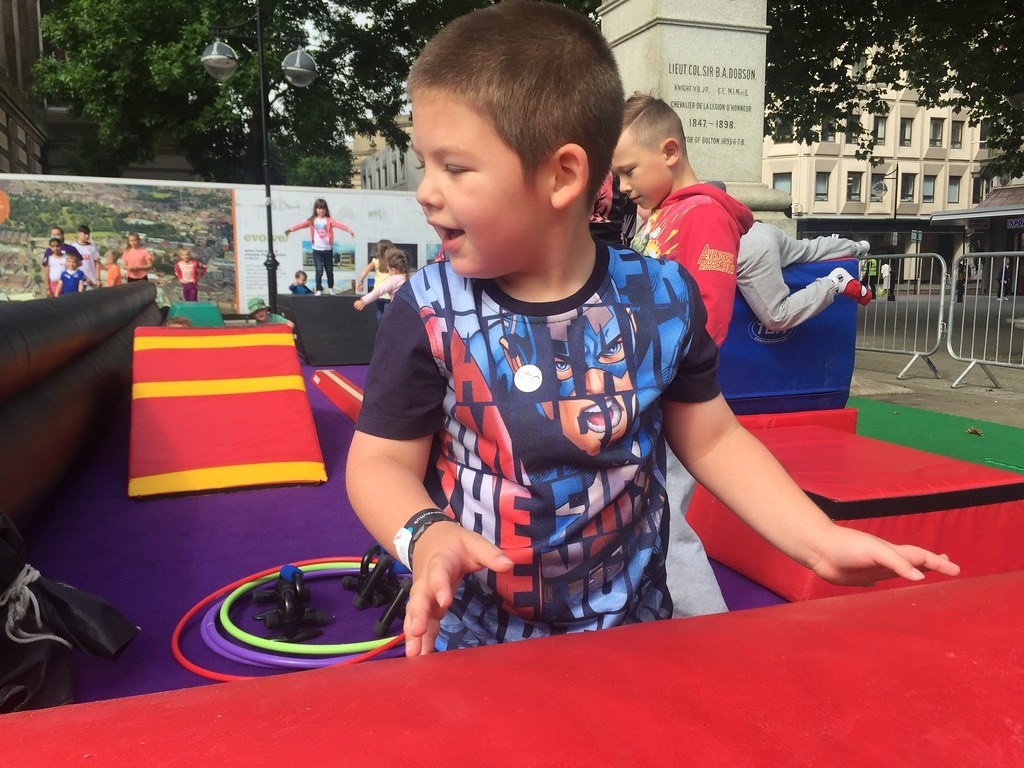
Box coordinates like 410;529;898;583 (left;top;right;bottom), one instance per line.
996;258;1011;301
881;260;891;290
345;0;960;657
248;297;295;330
357;239;396;299
122;232;153;282
860;253;877;300
174;248;208;301
42;225;121;297
609;95;754;619
636;182;872;332
290;270;314;295
285;199;356;295
354;249;411;311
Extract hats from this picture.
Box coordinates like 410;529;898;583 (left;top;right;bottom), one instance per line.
247;297;271;316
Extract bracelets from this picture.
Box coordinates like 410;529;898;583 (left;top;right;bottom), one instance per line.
393;508;460;571
360;281;363;284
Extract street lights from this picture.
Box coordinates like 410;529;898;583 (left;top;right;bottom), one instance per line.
200;8;319;313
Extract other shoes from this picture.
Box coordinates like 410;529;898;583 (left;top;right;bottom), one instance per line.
314;290;322;296
329;287;335;296
